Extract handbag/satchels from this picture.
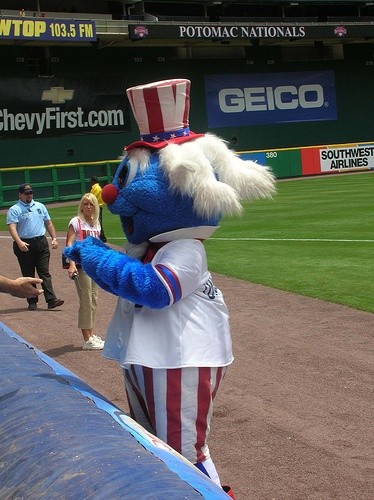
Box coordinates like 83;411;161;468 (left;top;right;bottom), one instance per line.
61;252;82;270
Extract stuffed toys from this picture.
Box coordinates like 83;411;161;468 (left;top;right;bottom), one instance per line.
67;78;278;498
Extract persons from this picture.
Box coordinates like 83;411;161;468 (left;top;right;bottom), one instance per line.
7;182;65;311
0;265;45;310
19;8;45;17
86;172;106;250
64;192;103;351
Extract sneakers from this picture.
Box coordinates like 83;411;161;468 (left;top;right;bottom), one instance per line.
83;335;104;349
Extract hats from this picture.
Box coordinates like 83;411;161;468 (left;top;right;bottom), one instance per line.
19;184;33;192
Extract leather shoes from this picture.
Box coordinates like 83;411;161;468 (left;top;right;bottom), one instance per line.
28;303;37;309
48;299;64;308
